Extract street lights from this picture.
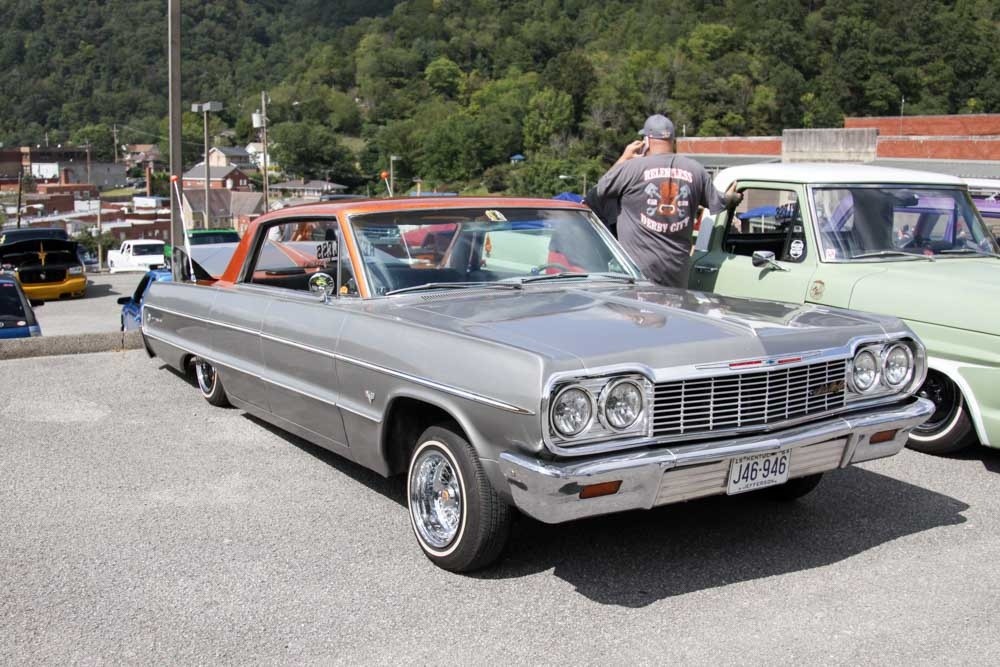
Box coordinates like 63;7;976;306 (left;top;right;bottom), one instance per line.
560;174;586;197
390;156;403;196
191;102;224;229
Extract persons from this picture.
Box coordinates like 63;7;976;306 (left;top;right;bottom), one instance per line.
593;113;744;293
545;186;624;276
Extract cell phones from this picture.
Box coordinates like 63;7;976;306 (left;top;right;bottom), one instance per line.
640;136;649;154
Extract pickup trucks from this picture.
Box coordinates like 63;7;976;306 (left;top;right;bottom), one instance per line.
107;239;167;275
478;161;1000;456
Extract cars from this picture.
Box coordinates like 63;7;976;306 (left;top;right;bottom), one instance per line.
0;225;100;341
736;179;1000;256
181;190;584;281
143;194;936;576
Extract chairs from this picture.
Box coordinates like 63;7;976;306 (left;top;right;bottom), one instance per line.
372;260;461;291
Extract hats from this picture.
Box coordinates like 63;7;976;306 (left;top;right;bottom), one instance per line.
638;114;675;139
